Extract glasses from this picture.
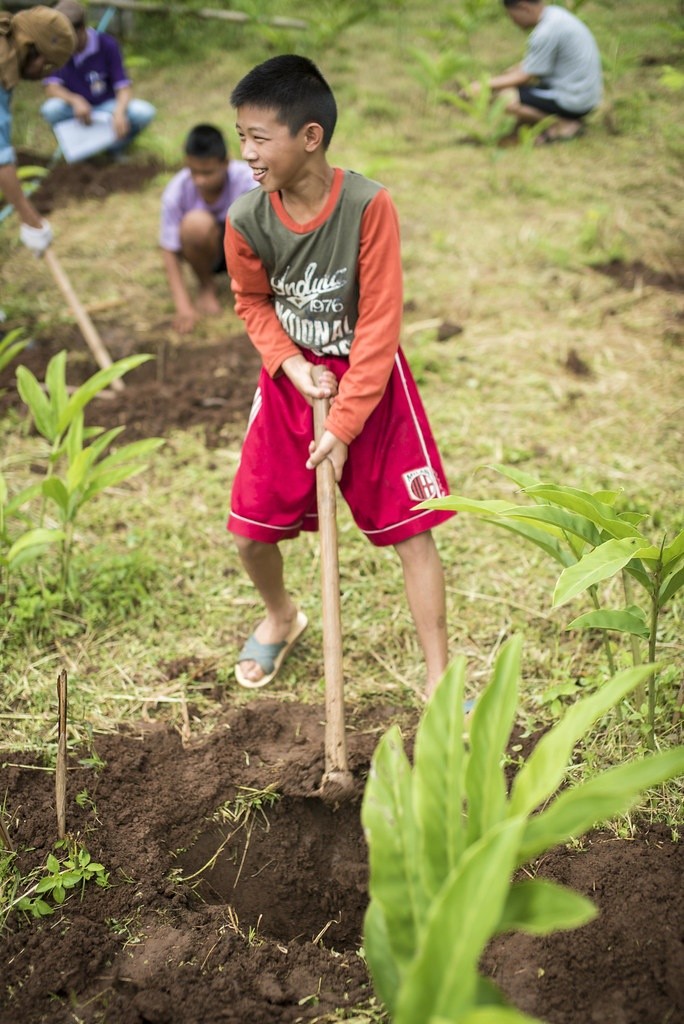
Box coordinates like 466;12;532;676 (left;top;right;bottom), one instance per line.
39;63;58;78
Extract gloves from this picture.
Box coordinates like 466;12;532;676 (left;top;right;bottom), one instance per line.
19;218;55;260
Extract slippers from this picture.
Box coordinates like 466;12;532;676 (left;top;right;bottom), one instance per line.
234;610;308;689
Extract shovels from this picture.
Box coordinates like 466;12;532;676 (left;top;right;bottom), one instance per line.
283;364;365;802
43;249;129;400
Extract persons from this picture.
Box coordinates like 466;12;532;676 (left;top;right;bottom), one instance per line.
154;51;479;722
458;0;605;139
160;124;262;329
0;0;159;259
